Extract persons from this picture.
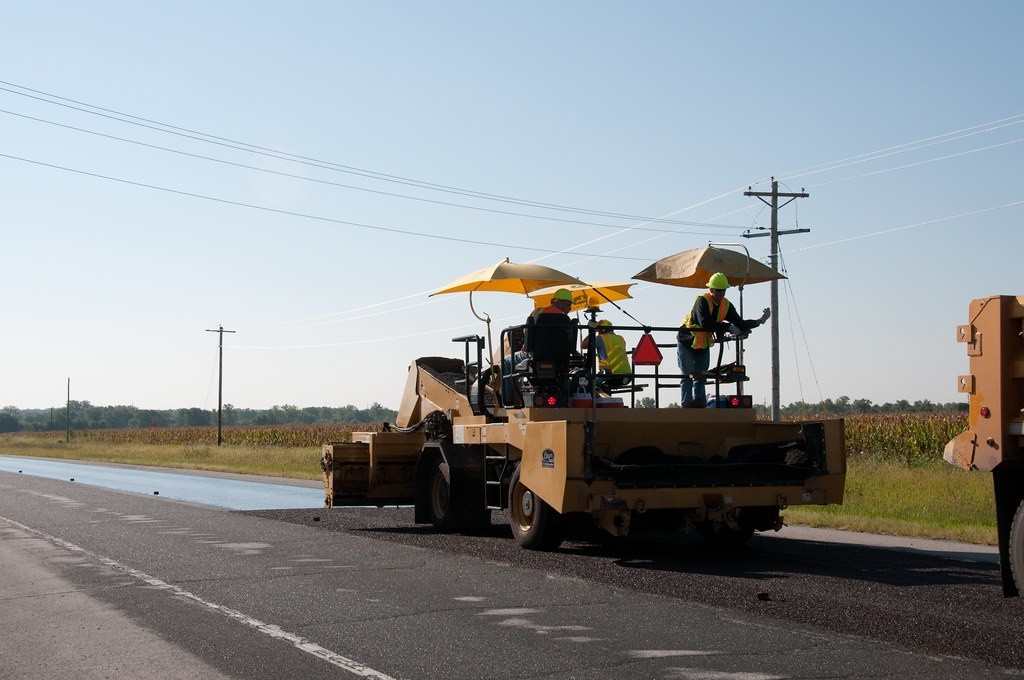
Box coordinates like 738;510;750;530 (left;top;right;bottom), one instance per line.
676;272;770;408
502;288;576;407
581;319;632;398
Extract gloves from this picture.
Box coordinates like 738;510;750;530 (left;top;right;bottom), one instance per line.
728;325;740;334
758;308;771;324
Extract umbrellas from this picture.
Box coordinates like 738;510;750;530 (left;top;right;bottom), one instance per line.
427;257;638;314
631;240;788;396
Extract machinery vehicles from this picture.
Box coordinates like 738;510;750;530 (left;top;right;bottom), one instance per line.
320;296;847;553
942;295;1023;602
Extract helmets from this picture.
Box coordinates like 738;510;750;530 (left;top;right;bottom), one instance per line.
597;320;612;326
552;289;574;304
706;272;730;289
